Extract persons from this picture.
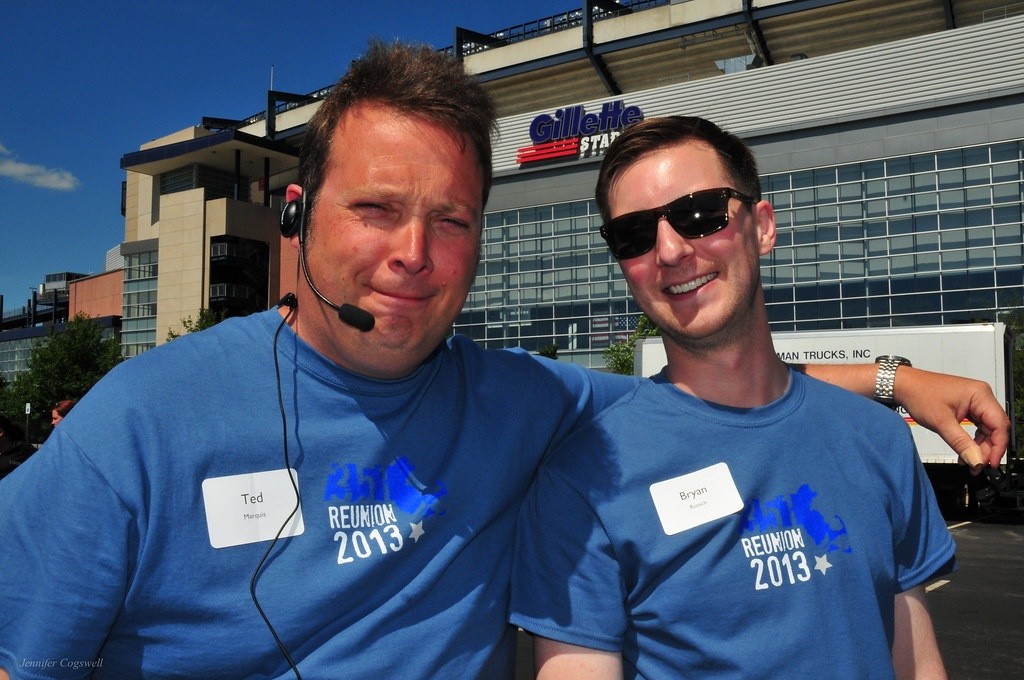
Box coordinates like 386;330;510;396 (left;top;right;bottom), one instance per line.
0;43;1010;680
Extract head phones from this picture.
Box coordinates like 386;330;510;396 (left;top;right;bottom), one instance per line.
280;190;307;238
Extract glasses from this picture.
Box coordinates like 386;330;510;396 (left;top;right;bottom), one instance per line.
599;187;758;261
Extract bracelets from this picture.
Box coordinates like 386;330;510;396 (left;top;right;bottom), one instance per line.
874;356;911;410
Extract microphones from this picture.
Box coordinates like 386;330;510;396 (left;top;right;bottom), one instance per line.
299;229;375;332
